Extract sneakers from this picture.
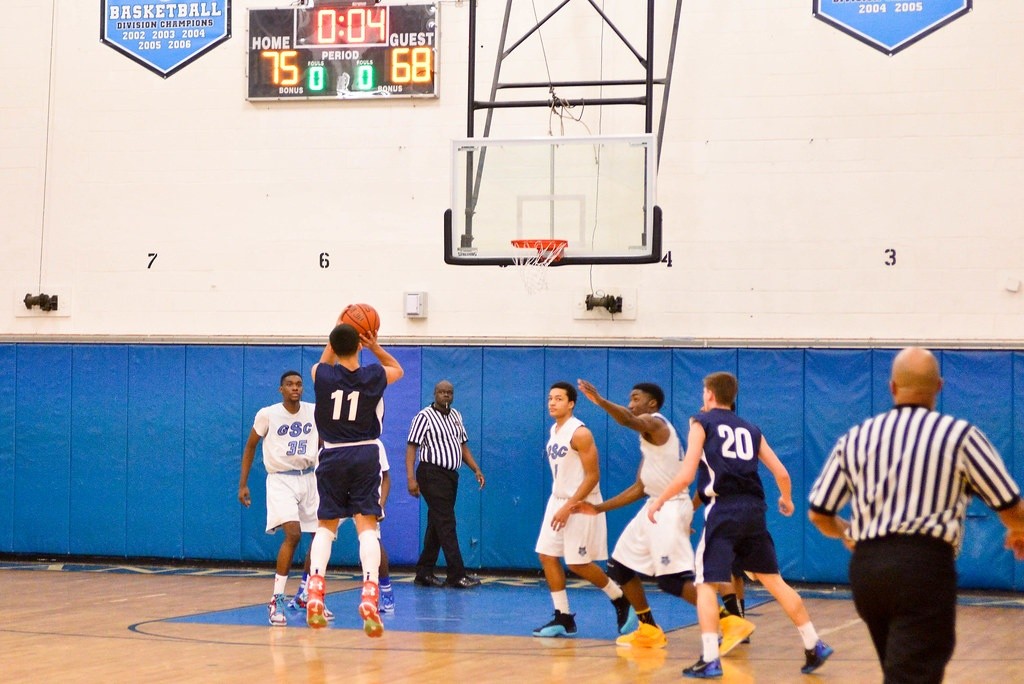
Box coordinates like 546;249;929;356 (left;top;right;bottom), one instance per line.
379;591;394;613
533;609;577;637
307;593;329;629
268;594;287;626
683;655;723;678
610;590;634;633
800;640;833;673
718;614;756;656
358;597;384;638
288;597;300;609
616;620;668;647
617;647;669;674
297;593;335;621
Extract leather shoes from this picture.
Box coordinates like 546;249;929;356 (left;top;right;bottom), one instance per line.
447;576;480;588
414;572;445;586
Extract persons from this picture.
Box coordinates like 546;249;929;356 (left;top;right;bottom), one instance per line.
647;372;833;680
568;378;757;657
307;304;404;638
239;370;335;626
807;346;1024;684
289;395;395;614
532;382;635;638
405;379;484;588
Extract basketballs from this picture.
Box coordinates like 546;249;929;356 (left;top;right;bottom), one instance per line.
341;303;380;342
